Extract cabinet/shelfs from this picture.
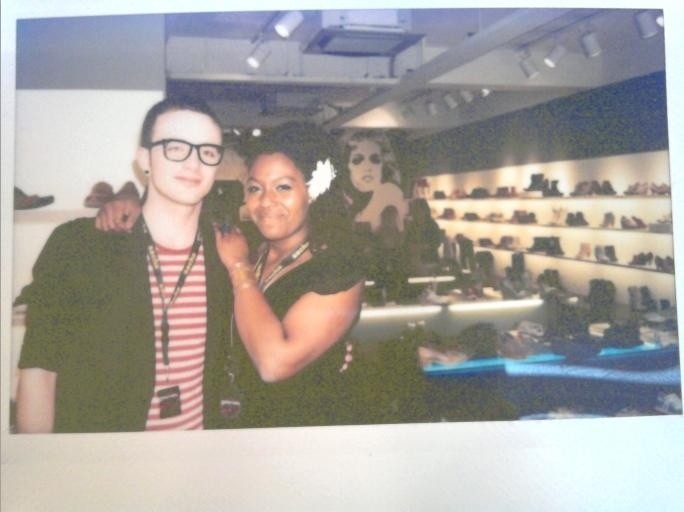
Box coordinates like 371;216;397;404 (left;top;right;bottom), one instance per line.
425;189;677;335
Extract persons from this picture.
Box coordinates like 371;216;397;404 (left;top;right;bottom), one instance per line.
15;96;355;431
336;129;409;230
93;121;365;426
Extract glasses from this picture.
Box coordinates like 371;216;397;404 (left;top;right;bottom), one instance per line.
147;138;225;166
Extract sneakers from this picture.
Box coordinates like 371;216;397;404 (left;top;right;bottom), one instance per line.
85;181;141;208
654;386;683;413
13;186;54;210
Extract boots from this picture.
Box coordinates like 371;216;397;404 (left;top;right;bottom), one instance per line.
367;240;674;366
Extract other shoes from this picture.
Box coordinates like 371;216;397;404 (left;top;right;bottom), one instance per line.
478;234;675;273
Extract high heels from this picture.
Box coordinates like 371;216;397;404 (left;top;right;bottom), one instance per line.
432;175;670;200
437;207;673;234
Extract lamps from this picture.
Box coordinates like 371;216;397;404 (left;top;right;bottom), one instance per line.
399;87;491;129
246;11;306;70
516;9;659;81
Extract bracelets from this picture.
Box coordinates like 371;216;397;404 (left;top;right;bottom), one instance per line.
228;262;256;293
